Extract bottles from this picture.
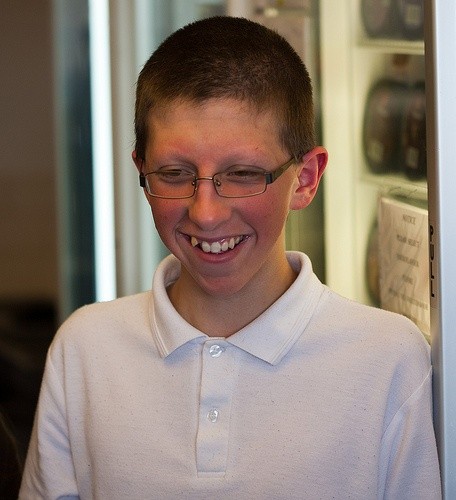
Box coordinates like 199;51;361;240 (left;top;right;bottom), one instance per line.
363;53;408;175
397;1;425;40
361;0;397;38
397;78;427;181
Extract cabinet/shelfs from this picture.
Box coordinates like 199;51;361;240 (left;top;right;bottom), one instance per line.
358;36;429;201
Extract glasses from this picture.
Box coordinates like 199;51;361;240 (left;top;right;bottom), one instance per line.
135;153;296;199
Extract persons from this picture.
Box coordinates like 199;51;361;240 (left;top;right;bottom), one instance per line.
16;15;443;500
